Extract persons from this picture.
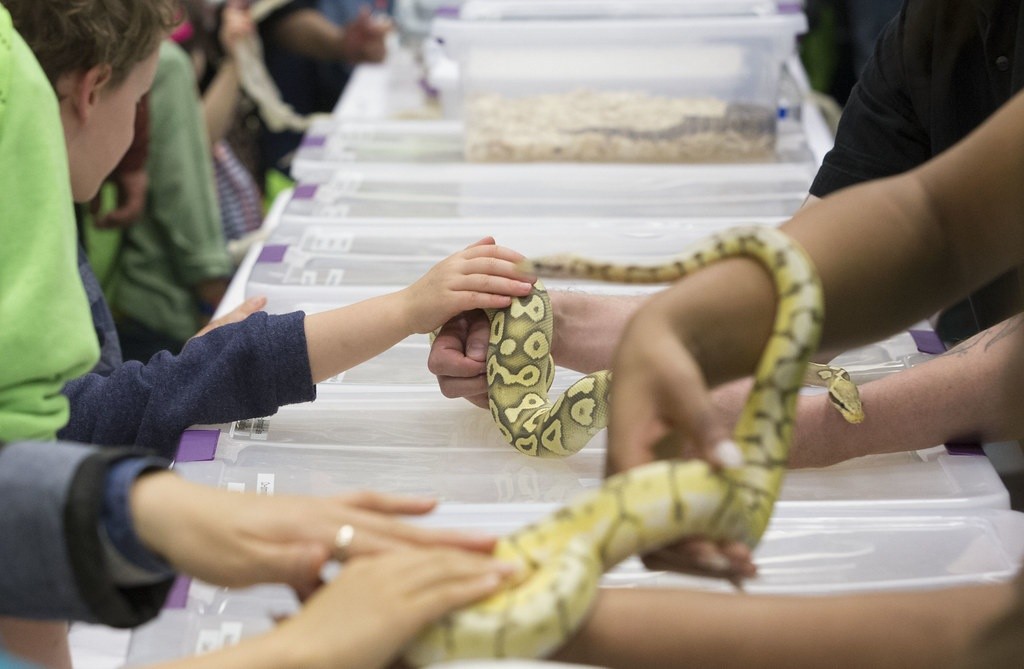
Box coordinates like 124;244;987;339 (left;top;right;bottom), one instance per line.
5;1;539;456
1;436;526;668
544;93;1024;668
427;0;1023;466
0;2;97;668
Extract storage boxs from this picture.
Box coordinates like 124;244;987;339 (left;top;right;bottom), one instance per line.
130;0;1024;669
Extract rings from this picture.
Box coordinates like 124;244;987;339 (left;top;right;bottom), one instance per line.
332;520;358;557
